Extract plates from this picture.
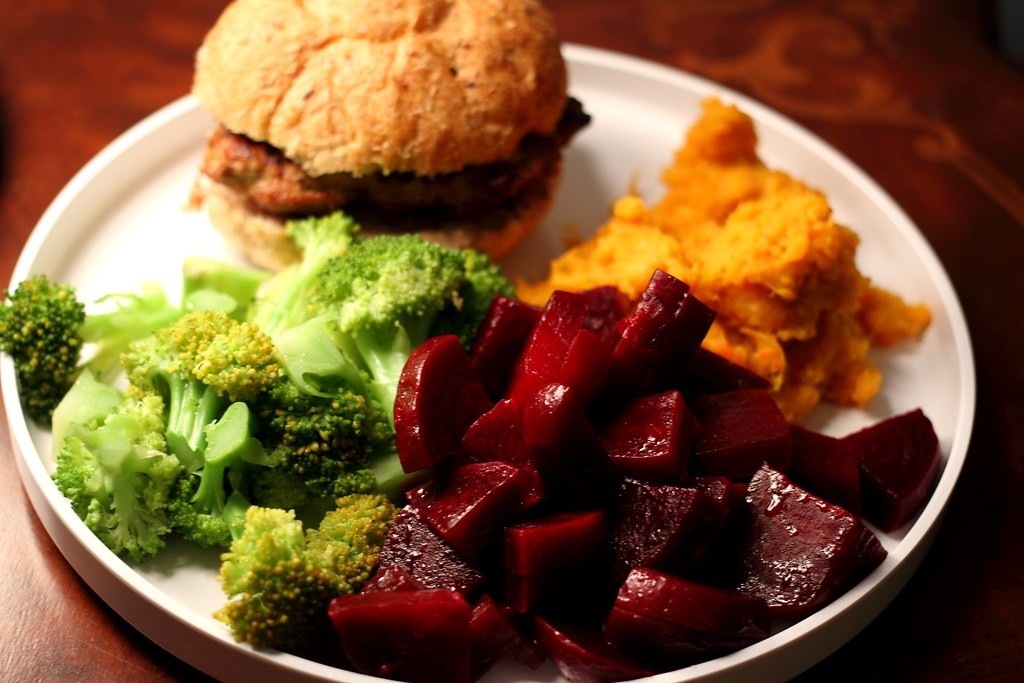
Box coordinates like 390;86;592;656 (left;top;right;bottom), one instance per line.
0;37;976;683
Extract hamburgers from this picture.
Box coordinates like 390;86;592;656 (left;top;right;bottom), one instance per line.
191;0;593;263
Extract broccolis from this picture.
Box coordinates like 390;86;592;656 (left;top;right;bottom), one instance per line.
1;208;524;646
